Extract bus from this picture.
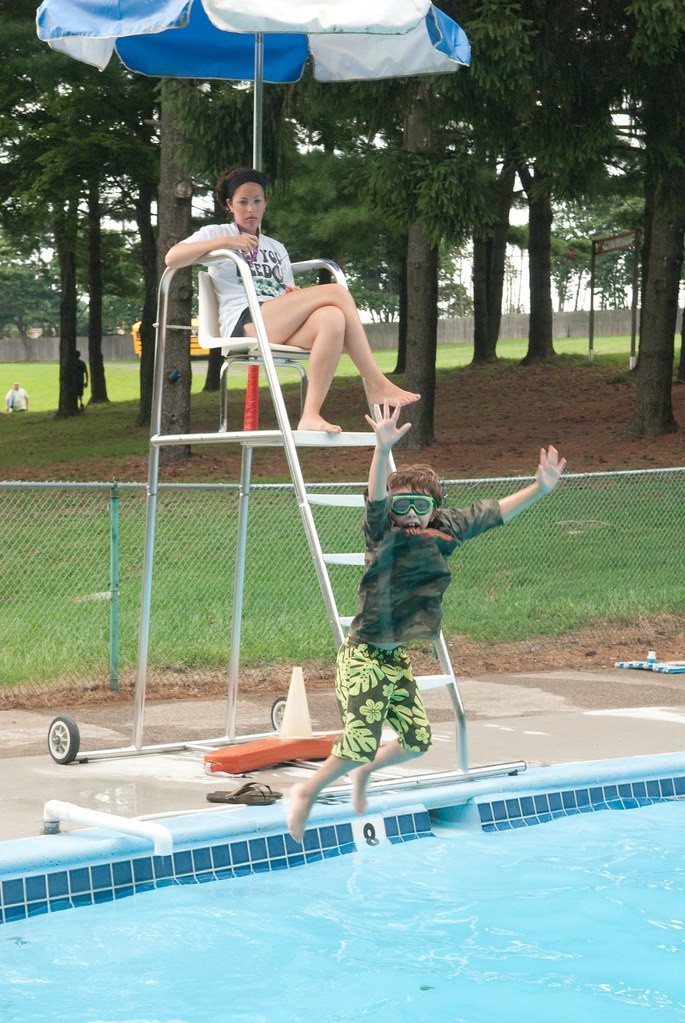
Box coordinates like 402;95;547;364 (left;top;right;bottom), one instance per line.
132;318;211;360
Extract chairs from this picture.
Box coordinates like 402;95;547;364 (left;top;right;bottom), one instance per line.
196;272;316;432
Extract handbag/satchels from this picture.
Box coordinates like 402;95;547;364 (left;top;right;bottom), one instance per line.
8;390;14;408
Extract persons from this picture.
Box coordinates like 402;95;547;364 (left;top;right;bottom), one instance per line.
163;167;421;433
5;383;29;412
288;401;569;842
74;350;88;410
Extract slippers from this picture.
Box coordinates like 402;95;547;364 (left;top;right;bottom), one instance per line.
214;782;282;798
206;787;276;805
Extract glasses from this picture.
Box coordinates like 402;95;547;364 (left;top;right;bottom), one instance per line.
390;493;439;516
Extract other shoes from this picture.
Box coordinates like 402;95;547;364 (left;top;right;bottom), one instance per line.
80;404;84;411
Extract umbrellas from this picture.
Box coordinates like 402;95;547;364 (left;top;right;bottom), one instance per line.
34;0;470;171
